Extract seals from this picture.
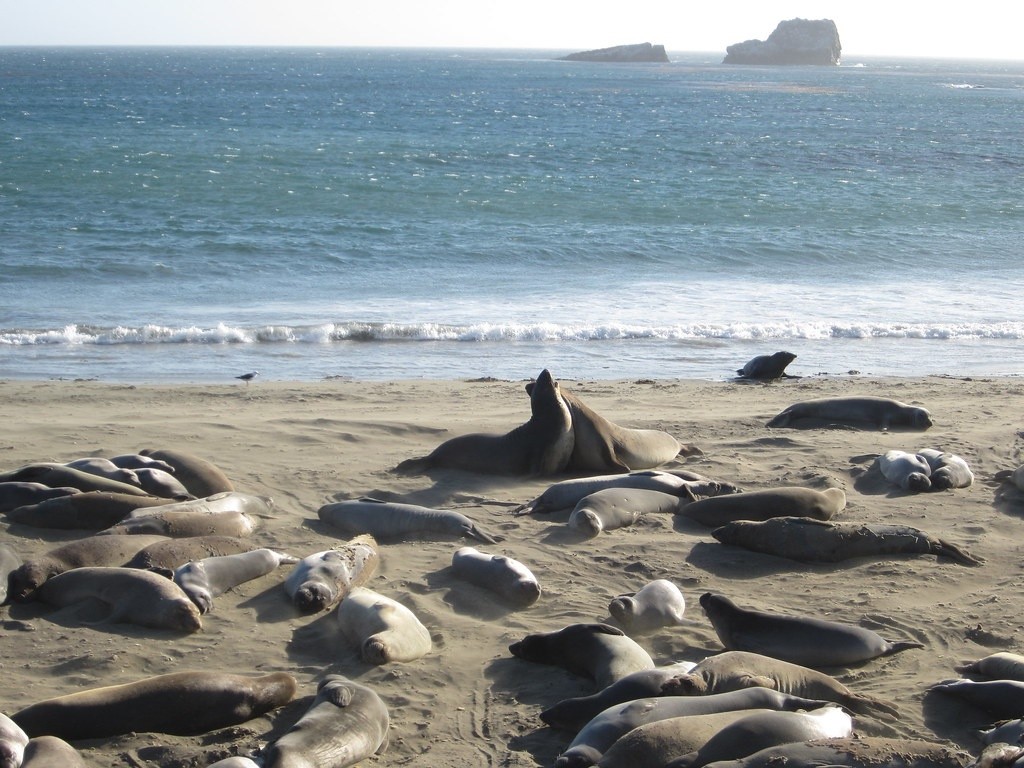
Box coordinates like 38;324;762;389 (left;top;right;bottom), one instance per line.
734;351;802;380
389;368;576;481
1;397;1023;767
523;383;704;477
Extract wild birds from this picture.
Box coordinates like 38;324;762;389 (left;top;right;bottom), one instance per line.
234;370;261;386
